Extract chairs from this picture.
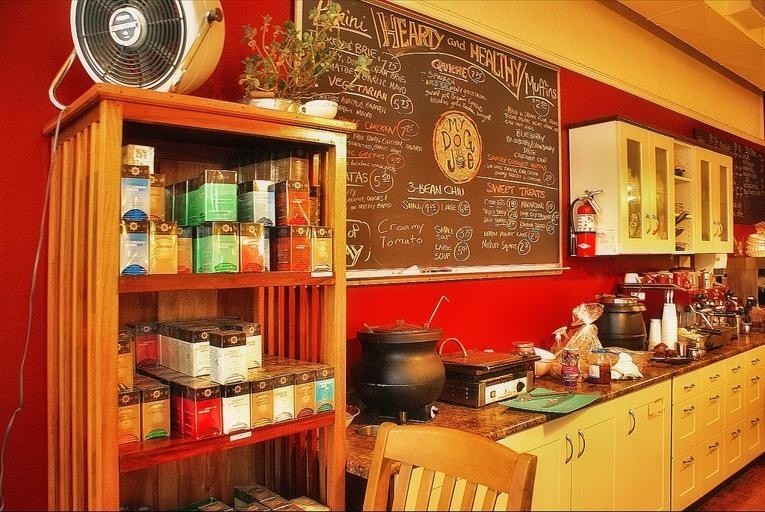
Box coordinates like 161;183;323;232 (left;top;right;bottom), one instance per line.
362;423;537;512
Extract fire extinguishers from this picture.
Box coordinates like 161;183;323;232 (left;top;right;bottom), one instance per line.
568;189;604;258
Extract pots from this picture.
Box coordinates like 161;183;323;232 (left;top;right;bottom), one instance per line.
352;319;447;423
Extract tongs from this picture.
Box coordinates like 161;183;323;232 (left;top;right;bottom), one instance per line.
517;390;575;406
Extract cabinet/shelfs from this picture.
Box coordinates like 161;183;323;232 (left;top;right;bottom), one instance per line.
346;435;516;512
672;343;765;512
516;398;618;511
619;379;671;512
566;114;674;255
43;84;358;512
673;132;696;255
696;140;734;253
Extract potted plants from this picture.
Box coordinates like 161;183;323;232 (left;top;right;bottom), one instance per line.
237;0;373;112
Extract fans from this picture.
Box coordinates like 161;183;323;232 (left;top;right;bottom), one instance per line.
47;0;224;110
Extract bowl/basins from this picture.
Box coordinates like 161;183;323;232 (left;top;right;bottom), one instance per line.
533;347;555;378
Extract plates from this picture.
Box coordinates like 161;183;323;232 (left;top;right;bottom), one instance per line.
497;387;601;414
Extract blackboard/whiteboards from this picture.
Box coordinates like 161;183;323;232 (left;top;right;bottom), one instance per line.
294;0;562;286
692;128;765;225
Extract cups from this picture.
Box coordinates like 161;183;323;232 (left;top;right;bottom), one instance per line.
299;99;337;119
656;271;713;289
623;272;641;284
675;342;688;356
740;322;752;333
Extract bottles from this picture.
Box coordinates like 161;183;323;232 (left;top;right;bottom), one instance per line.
511;340;536;386
648;318;661;352
661;289;678;350
560;347;580;388
